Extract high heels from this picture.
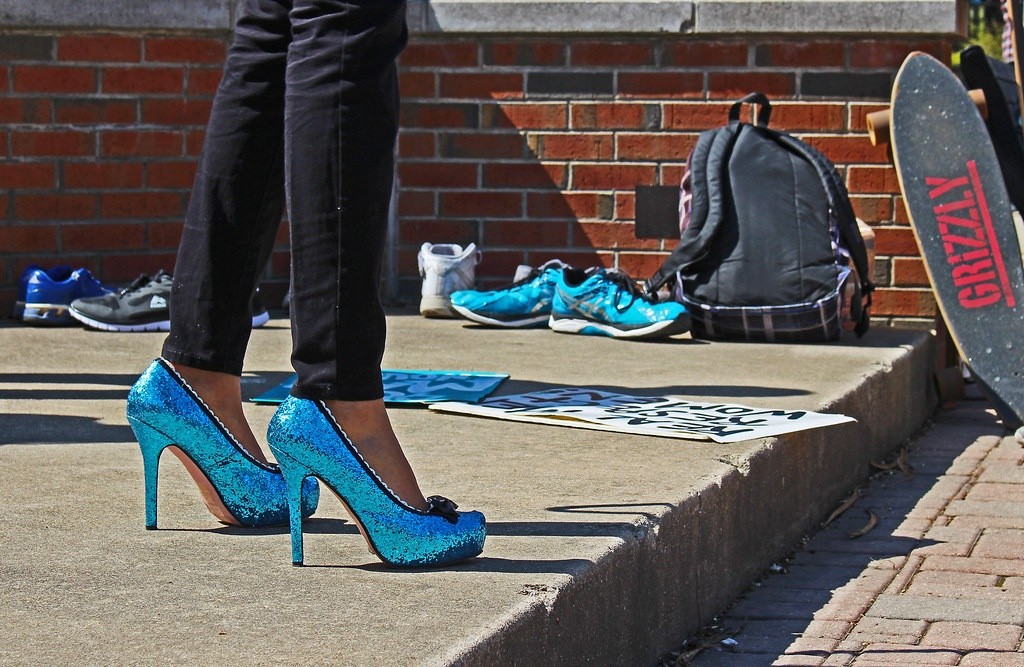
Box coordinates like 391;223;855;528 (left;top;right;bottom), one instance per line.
265;394;486;569
126;358;318;530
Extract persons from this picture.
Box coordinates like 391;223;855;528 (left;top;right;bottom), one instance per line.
126;0;486;570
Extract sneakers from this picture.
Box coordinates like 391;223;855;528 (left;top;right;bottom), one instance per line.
15;262;123;324
449;259;598;328
415;241;483;318
70;269;269;330
548;265;688;339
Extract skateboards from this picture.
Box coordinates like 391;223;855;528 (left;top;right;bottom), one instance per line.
863;48;1024;434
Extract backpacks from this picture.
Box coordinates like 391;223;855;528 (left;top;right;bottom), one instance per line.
640;93;876;343
